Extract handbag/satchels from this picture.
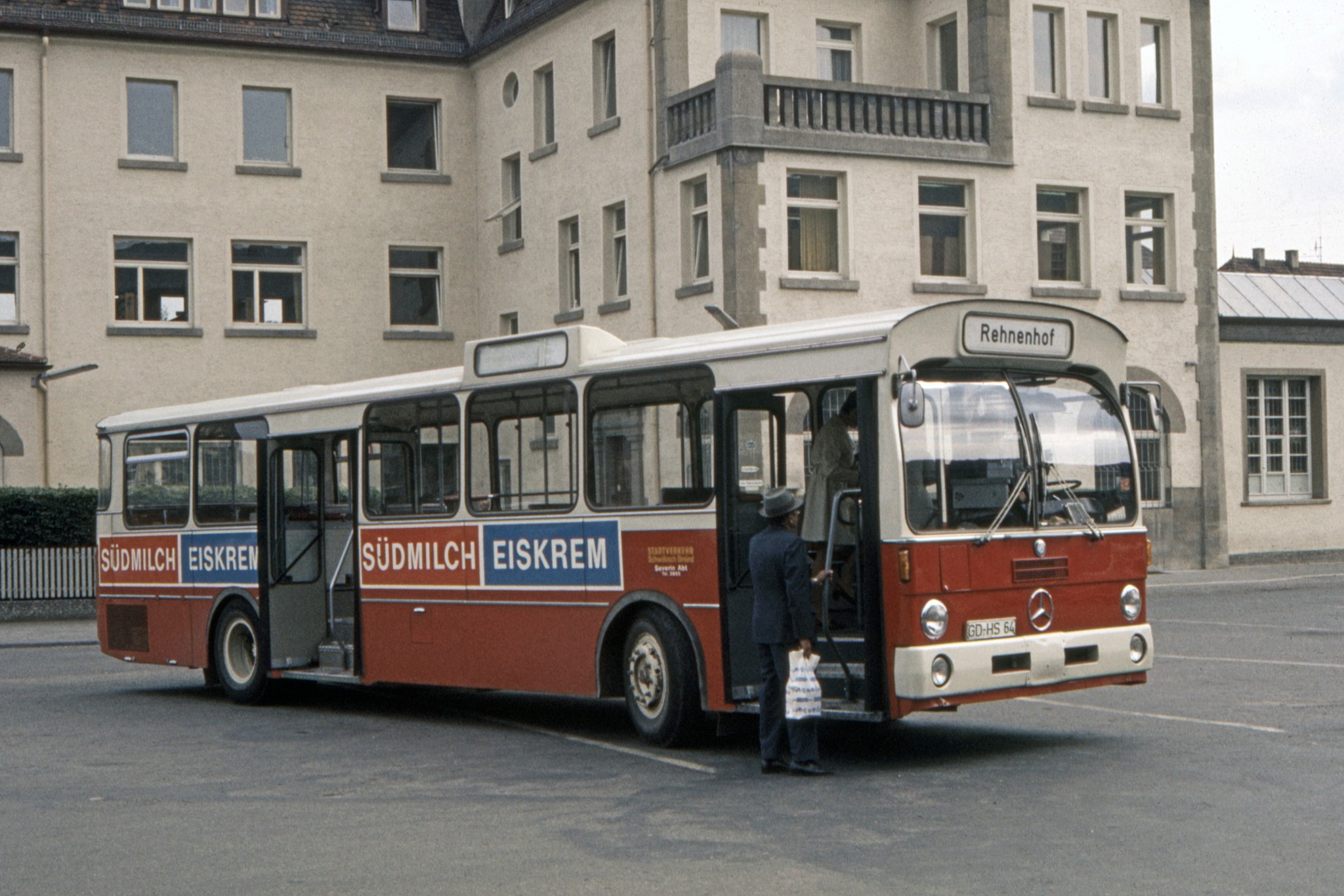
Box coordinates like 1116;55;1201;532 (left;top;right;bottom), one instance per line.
784;649;822;720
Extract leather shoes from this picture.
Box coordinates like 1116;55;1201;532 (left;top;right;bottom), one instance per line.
814;616;846;630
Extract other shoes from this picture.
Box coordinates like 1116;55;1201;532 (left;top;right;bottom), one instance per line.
787;759;834;776
761;760;788;772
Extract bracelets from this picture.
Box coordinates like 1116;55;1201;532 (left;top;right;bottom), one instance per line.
813;578;818;585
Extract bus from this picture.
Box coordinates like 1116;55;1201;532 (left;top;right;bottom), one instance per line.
93;298;1158;751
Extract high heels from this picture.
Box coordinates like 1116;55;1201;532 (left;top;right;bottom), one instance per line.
832;582;857;602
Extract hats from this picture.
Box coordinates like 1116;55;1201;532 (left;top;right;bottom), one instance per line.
759;487;804;517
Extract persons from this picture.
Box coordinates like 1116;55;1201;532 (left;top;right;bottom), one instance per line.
747;486;832;774
800;390;860;633
902;427;936;530
